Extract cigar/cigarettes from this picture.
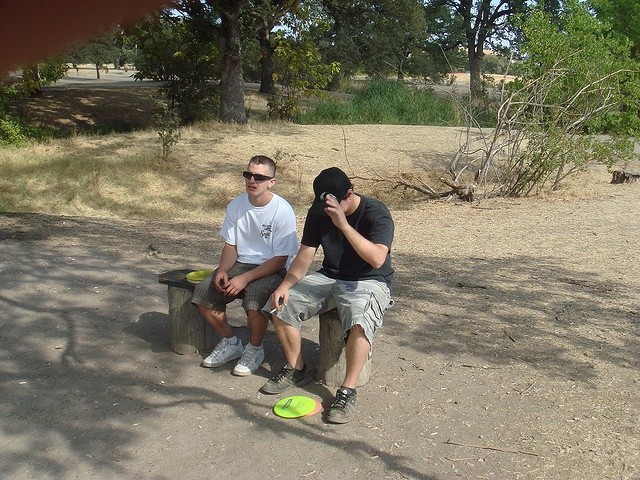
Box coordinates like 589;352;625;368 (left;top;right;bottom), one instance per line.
270;305;282;314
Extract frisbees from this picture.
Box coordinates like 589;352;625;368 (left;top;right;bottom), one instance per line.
186;270;213;285
304;399;322;417
274;396;316;418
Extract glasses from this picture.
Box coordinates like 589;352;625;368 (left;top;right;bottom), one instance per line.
242;171;276;181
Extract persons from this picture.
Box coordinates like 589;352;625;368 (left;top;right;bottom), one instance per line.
260;166;395;424
190;155;299;377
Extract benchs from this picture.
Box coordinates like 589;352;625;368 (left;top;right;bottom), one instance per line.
158;268;373;388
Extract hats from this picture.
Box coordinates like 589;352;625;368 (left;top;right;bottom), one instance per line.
310;166;349;217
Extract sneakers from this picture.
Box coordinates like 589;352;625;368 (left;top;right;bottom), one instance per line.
233;345;265;377
260;363;313;395
327;387;358;423
202;336;244;367
